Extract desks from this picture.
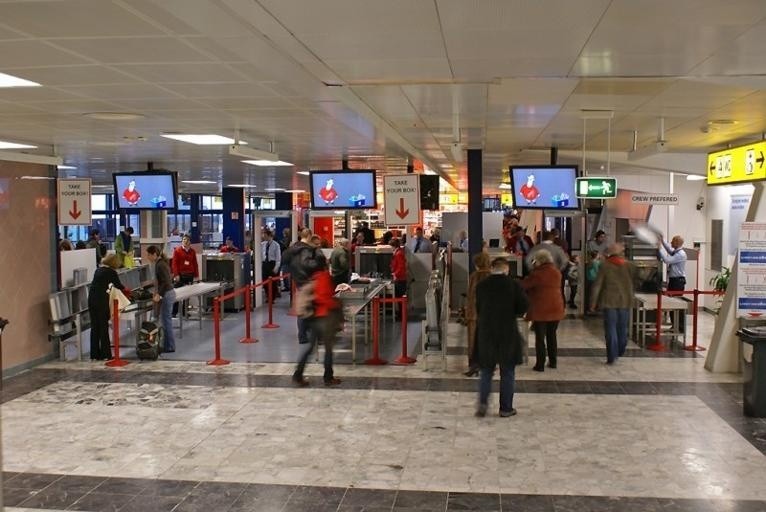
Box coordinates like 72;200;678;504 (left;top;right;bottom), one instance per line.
122;279;232;338
315;275;400;365
628;293;689;351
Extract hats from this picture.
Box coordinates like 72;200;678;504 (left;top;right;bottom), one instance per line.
603;243;625;257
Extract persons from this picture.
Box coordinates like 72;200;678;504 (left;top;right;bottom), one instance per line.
475;257;529;418
222;227;325;342
58;228;134;268
88;254;125;360
172;235;199;318
328;221;441;322
293;254;341;386
511;249;565;372
464;254;492;377
459;215;607;317
657;233;687;334
590;242;635;364
146;246;176;352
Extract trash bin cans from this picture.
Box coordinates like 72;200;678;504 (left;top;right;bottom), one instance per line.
734;325;766;418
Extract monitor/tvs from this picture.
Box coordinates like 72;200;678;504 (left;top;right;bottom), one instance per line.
310;169;377;210
112;169;179;211
508;165;582;211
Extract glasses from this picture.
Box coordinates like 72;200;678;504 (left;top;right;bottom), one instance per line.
531;259;536;267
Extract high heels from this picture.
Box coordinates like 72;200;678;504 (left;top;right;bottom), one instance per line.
99;354;112;361
90;354;98;361
462;367;480;378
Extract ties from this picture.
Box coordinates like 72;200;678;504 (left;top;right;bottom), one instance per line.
667;250;675;275
266;243;270;262
414;240;421;253
519;238;527;255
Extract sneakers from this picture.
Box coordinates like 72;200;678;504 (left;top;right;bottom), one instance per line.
546;363;557;368
161;348;176;353
325;377;342;387
618;350;625;357
499;409;517;418
605;358;617;364
532;366;544;371
292;375;312;386
475;405;488;418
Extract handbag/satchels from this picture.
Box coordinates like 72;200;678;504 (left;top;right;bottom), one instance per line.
124;255;135;269
135;321;161;361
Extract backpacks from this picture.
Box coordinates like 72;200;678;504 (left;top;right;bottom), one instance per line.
294;281;315;319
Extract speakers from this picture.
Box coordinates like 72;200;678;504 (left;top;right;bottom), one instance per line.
420;174;439;211
